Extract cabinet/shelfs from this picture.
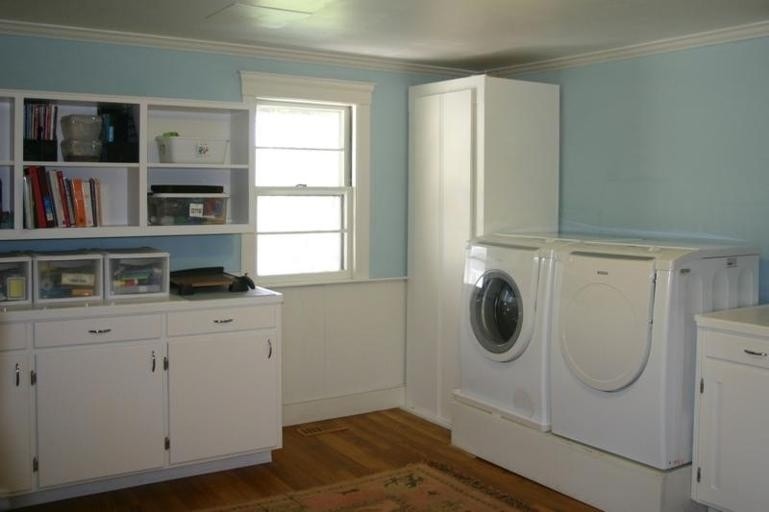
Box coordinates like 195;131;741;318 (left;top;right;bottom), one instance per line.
0;309;34;512
690;304;769;512
405;74;560;432
34;301;167;506
166;295;285;481
0;89;256;240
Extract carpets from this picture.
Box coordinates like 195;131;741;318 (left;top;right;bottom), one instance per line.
192;455;540;512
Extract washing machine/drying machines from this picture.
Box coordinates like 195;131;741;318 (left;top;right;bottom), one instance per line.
548;239;765;473
448;229;552;432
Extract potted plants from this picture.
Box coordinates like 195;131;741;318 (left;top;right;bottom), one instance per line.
155;131;230;164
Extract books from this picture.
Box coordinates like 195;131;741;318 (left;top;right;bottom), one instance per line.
25;101;58;161
23;166;107;229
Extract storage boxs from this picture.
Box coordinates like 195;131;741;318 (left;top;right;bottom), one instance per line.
147;192;232;225
0;256;33;306
34;254;104;305
104;252;170;303
